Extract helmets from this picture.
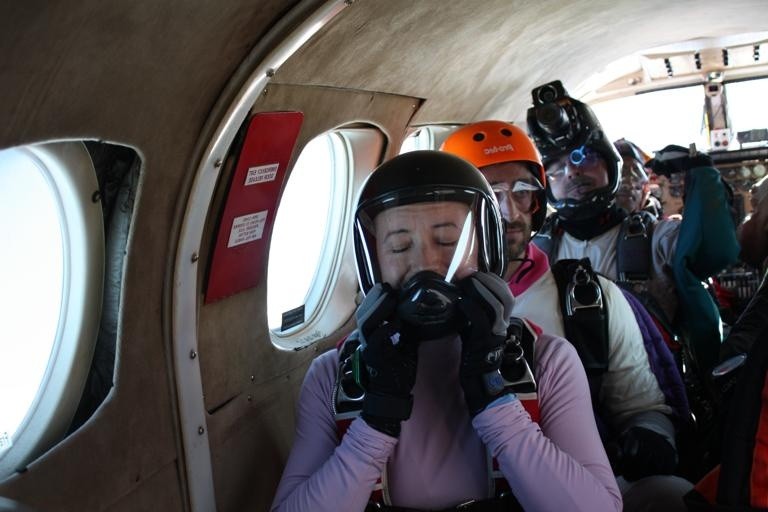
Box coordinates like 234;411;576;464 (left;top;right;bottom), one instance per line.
439;121;546;231
526;79;619;221
352;150;510;340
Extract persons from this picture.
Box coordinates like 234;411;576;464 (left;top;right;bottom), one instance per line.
270;80;768;510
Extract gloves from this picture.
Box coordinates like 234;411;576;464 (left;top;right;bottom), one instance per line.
355;282;419;437
454;272;515;422
621;426;675;483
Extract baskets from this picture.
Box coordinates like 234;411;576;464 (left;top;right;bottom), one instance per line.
714;261;759;308
